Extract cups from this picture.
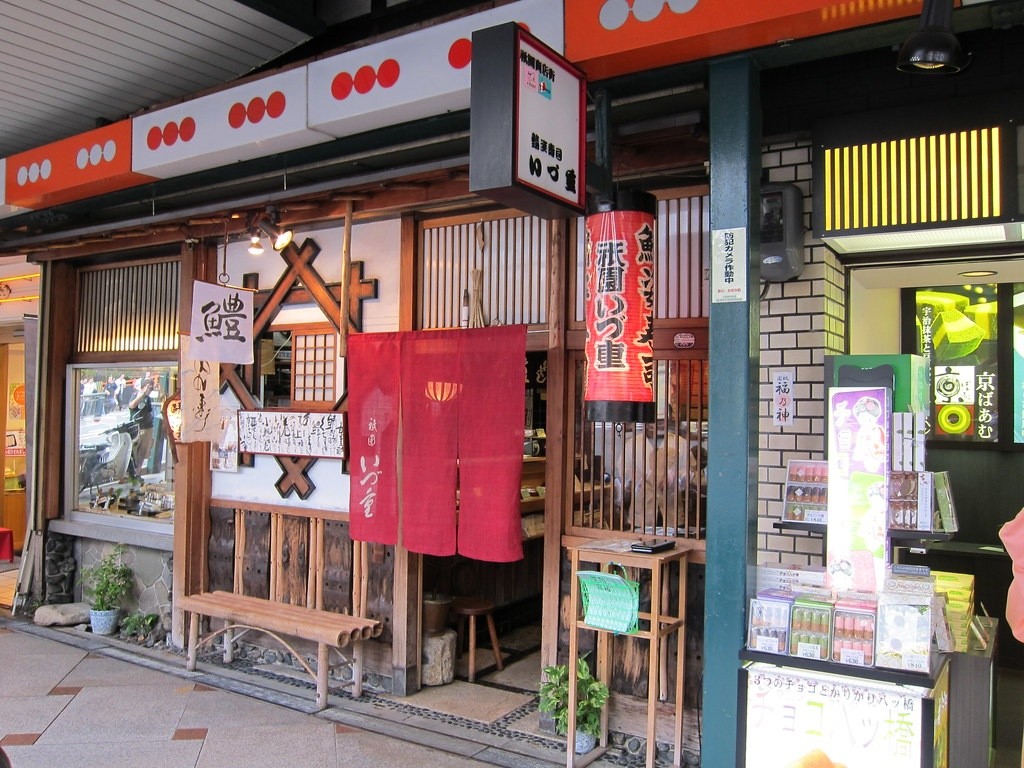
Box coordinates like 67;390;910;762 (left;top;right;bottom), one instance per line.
523;441;539;459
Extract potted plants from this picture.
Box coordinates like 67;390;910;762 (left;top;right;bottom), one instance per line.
75;542;133;637
539;659;610;755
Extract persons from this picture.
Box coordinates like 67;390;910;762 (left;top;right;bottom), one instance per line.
679;439;709;529
128;377;155;476
114;373;126;412
102;377;118;412
83;378;88;385
81;377;85;414
127;371;152;401
80;377;98;414
616;402;696;531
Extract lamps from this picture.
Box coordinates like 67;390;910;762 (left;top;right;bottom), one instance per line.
257;204;294;251
896;0;969;75
248;226;264;256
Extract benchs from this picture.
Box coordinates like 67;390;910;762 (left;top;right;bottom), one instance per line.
178;589;384;711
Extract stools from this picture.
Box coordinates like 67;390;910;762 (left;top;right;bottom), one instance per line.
449;594;506;682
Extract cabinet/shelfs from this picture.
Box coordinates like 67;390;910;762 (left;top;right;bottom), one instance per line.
567;537;694;767
453;448;614;553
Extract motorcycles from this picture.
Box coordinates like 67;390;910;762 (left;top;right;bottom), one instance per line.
77;402;145;493
